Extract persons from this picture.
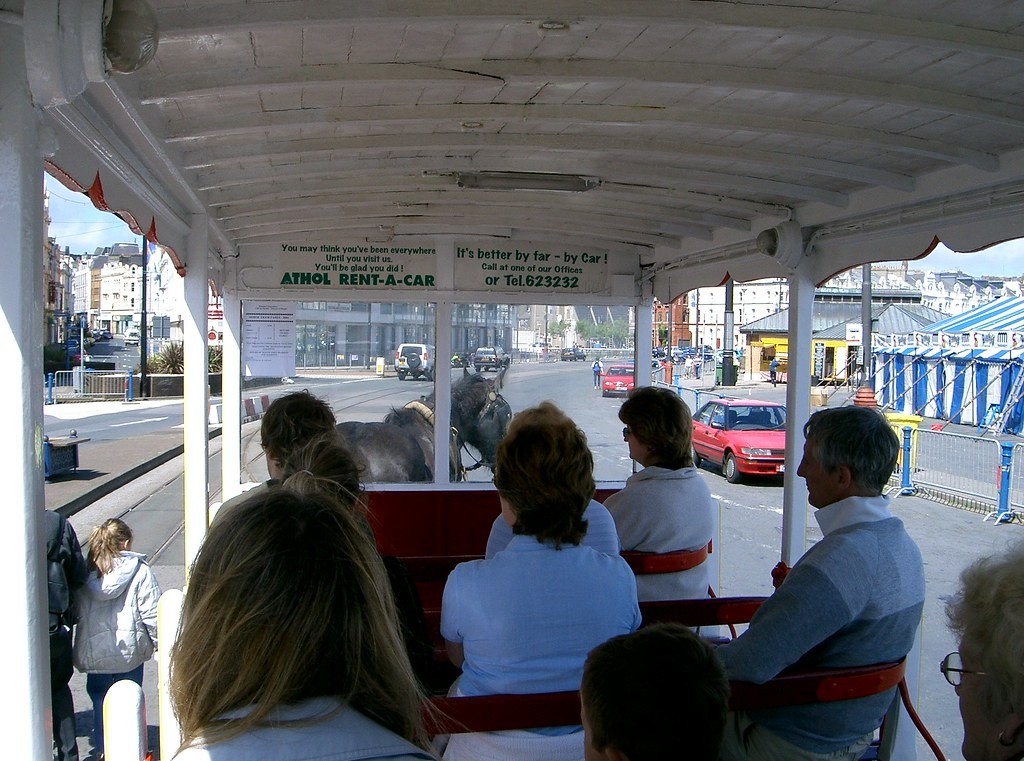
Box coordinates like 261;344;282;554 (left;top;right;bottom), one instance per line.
603;386;717;599
717;407;926;761
278;443;463;696
577;623;733;761
769;358;781;383
591;357;604;389
693;355;702;379
437;399;642;761
44;508;89;761
685;355;694;380
170;469;442;761
937;533;1024;760
260;387;335;483
75;518;166;761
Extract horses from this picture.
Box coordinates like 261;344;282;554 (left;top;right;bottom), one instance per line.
334;362;512;484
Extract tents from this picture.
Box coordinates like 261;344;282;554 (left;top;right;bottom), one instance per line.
871;293;1024;439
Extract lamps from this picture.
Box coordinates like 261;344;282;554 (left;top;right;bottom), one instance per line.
24;0;159;110
456;174;599;191
756;220;804;268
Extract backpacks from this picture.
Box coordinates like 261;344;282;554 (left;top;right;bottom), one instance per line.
47;560;74;643
593;362;600;372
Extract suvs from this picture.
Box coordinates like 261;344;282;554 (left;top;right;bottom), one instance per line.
474;346;510;371
394;344;434;380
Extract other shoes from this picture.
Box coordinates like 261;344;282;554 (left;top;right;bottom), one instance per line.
598;386;600;389
594;386;596;389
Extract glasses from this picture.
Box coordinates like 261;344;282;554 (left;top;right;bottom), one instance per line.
622;428;632;438
939;652;989;686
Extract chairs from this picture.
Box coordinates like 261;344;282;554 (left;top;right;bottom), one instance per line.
728;410;737;424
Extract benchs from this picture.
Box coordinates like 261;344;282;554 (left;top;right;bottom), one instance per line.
387;556;946;761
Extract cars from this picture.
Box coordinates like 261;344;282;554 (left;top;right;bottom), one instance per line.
692;397;787;484
600;365;636;398
62;328;141;361
561;348;587;361
625;344;741;381
